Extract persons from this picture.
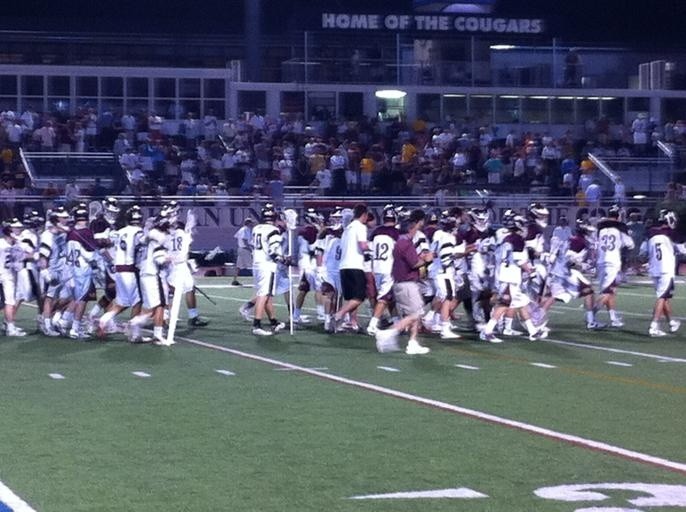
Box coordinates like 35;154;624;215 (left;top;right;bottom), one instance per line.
232;203;682;355
563;46;583;84
0;195;209;352
346;47;360;80
0;104;685;199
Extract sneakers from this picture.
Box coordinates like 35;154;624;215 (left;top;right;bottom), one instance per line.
610;319;625;327
421;312;462;339
668;320;680;333
187;316;210;329
36;315;91;340
94;309;174;346
238;304;253;322
292;312;300;323
316;313;364;334
5;326;26;337
405;340;430;355
647;327;667;337
251;325;273;336
587;321;606;329
475;323;550;343
374;330;387;354
1;321;24;331
366;326;379;336
271;322;286;332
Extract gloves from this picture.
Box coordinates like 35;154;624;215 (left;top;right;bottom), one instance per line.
282;254;298;267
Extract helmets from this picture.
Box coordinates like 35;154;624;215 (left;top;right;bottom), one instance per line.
608;202;623;221
500;209;516;225
260;201;325;224
0;203;89;235
576;217;595;237
526;203;550;228
507;214;528;230
100;197;182;226
657;208;677;229
423;205;489;230
329;203;411;222
